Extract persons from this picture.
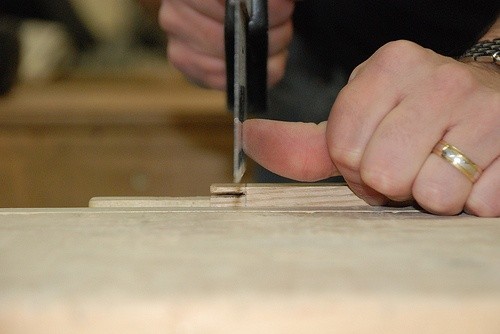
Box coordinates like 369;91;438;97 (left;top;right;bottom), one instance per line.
157;0;500;217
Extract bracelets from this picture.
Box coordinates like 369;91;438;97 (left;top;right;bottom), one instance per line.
457;37;500;66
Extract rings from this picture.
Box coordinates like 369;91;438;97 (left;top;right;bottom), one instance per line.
431;139;483;184
